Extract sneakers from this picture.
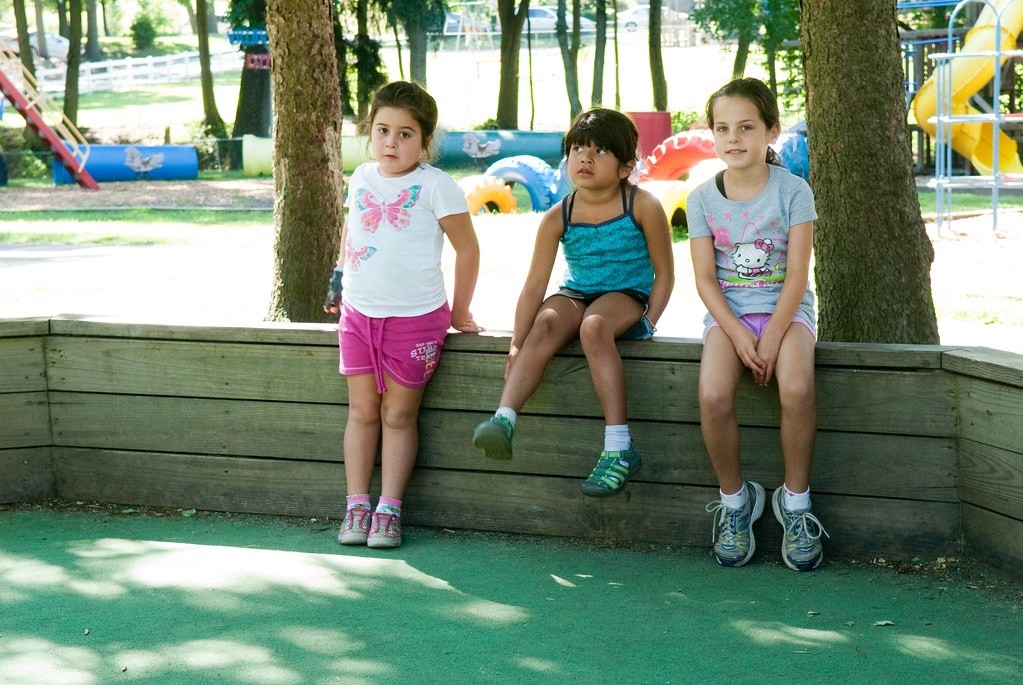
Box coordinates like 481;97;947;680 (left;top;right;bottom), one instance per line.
713;481;765;566
337;506;371;546
367;512;402;548
772;486;823;571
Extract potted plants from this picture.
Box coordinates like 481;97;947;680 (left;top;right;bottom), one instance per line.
225;0;270;46
236;43;271;70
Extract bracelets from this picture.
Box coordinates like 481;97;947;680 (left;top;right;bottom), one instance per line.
645;315;657;332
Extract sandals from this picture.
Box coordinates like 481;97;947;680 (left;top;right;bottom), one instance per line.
471;415;514;460
582;438;643;497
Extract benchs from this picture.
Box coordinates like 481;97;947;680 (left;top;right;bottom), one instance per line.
1;310;1022;588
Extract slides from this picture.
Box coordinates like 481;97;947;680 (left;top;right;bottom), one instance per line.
914;0;1022;176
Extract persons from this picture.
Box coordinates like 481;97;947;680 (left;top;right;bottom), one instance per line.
473;109;676;497
323;82;486;548
685;79;825;571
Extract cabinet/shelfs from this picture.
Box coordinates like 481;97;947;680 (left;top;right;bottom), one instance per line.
926;0;1023;238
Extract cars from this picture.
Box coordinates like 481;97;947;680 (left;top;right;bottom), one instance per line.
1;27;86;62
442;13;487;35
492;7;596;32
618;1;692;30
